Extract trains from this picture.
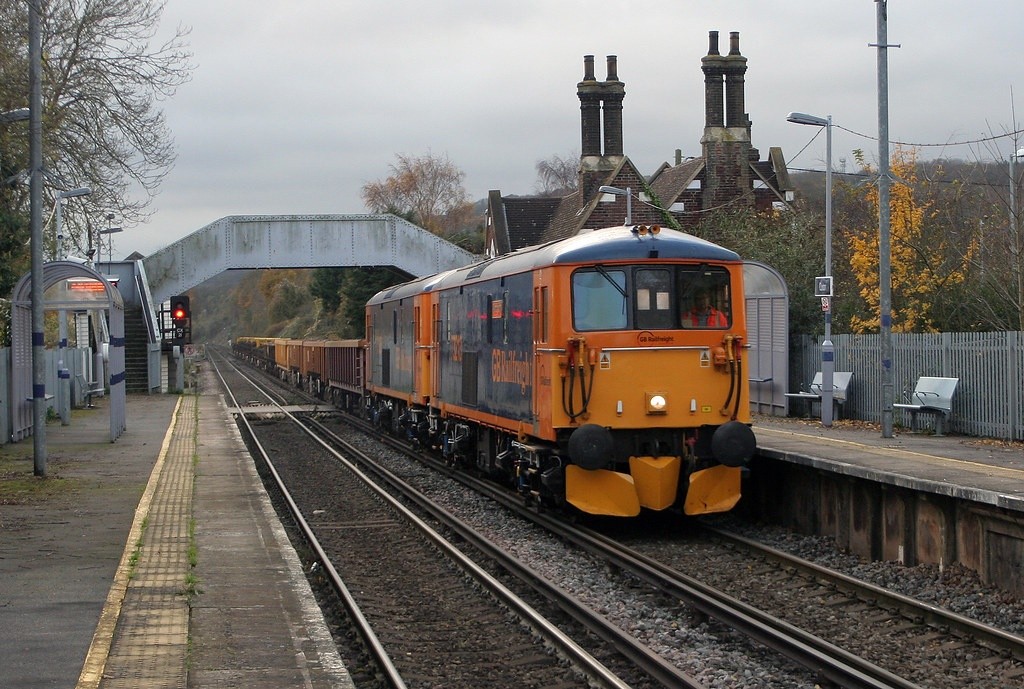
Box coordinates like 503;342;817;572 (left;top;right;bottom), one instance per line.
231;224;761;528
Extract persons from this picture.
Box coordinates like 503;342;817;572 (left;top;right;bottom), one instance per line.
681;290;727;328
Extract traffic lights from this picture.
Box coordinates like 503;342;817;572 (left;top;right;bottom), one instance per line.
170;294;190;320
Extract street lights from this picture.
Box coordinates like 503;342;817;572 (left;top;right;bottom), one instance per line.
97;227;124;270
786;110;835;429
56;187;91;417
599;186;632;226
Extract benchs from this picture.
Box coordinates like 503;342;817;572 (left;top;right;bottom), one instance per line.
76;374;106;409
784;371;854;419
893;376;959;436
